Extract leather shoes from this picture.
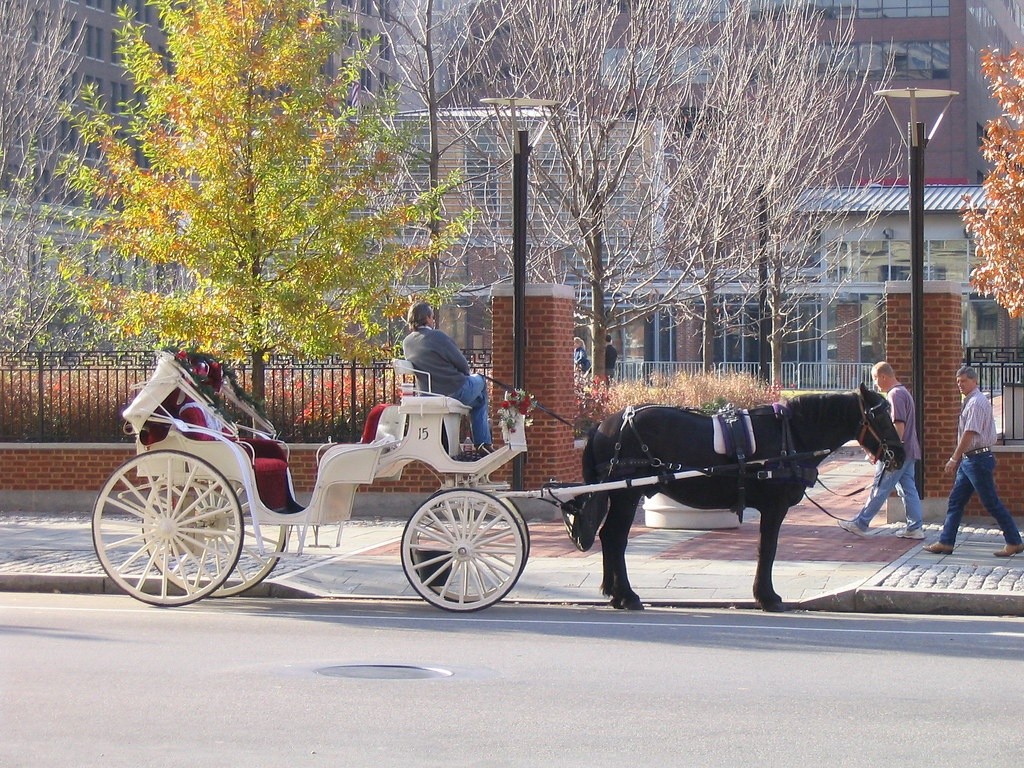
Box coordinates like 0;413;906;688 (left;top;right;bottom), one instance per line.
923;542;953;554
993;541;1023;556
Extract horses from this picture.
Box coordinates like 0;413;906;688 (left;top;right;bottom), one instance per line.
577;383;907;611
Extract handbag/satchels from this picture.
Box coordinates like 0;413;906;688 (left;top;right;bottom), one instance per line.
577;359;591;374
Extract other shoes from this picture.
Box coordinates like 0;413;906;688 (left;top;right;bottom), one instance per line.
476;444;495;456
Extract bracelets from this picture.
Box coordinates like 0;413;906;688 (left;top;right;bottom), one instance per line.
950;457;957;462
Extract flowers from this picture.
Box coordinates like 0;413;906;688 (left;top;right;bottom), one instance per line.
497;388;536;433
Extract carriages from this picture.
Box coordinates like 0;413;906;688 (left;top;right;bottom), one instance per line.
90;335;908;612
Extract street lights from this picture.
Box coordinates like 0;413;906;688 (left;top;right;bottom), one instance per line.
478;95;563;490
873;87;960;501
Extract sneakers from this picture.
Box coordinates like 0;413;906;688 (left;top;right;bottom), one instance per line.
895;527;924;540
838;519;866;538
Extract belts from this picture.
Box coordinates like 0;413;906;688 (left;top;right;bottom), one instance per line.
962;447;990;459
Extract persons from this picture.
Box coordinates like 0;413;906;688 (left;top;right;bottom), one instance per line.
837;361;924;540
605;335;617;378
403;302;495;457
923;366;1024;557
574;337;587;380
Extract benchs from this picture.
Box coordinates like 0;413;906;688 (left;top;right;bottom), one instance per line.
151;403;288;512
394;359;471;458
317;405;406;477
